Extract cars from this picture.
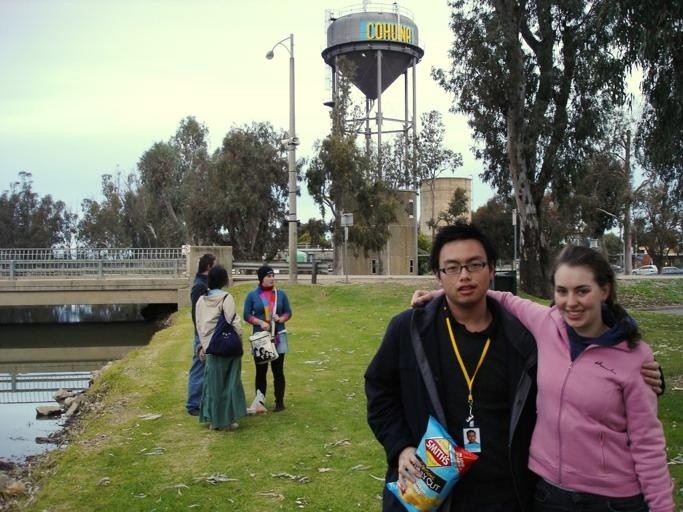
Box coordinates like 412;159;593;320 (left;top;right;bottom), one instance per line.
610;265;683;275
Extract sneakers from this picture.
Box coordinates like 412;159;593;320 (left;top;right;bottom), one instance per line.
189;409;199;416
273;401;285;412
210;422;239;432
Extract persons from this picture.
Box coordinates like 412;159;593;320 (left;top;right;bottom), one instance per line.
184;252;217;417
242;264;292;414
408;244;678;512
361;220;668;512
194;263;248;432
465;429;479;445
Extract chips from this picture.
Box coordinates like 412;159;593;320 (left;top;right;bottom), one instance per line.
396;480;442;510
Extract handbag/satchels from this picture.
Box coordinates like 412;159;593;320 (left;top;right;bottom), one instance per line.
247;331;278;366
203;312;243;357
246;390;269;417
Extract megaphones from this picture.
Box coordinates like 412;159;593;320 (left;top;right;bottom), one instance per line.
280;139;288;146
290;137;300;145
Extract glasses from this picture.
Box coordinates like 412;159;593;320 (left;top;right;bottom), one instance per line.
438;262;487;276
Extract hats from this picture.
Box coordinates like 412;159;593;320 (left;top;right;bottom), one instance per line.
257;266;273;281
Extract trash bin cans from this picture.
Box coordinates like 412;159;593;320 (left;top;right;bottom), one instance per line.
494;270;517;297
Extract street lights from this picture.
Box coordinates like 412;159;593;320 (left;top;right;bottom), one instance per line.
266;33;300;283
